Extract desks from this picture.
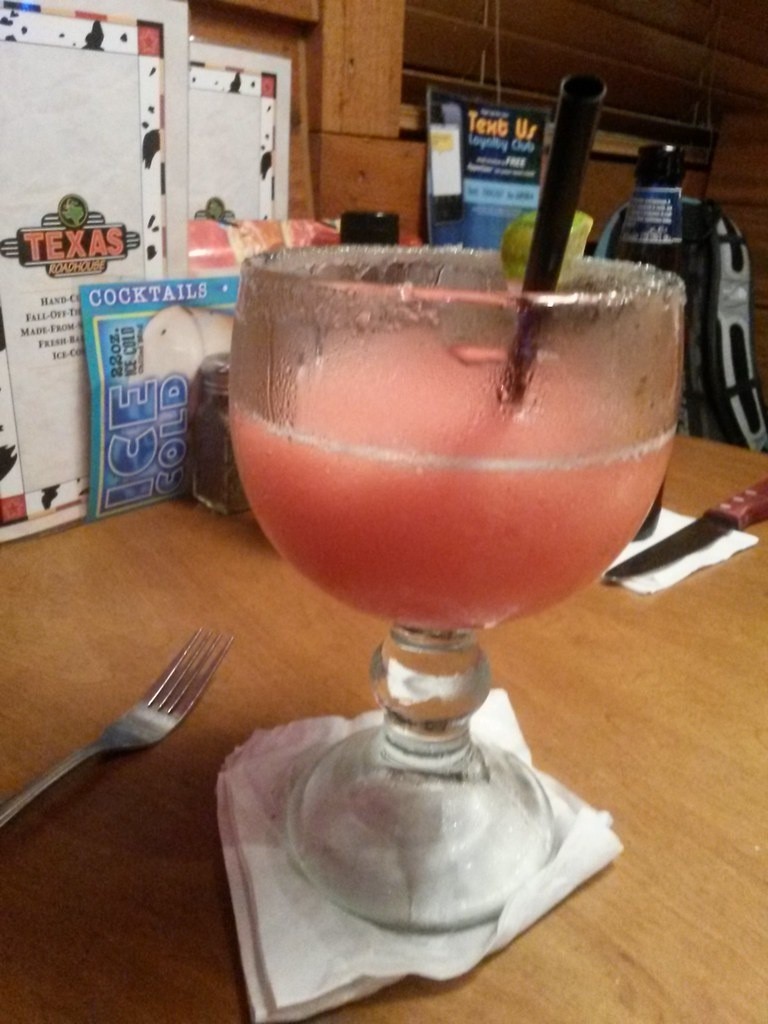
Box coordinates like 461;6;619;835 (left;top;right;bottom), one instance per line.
0;429;768;1023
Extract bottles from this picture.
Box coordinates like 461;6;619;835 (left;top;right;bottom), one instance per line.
614;140;685;281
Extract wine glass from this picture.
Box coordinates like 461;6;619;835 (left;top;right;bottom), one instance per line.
228;243;688;932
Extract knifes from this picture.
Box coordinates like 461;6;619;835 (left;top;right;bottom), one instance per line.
603;475;768;583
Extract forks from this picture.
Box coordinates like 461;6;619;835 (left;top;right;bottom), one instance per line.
1;621;234;828
